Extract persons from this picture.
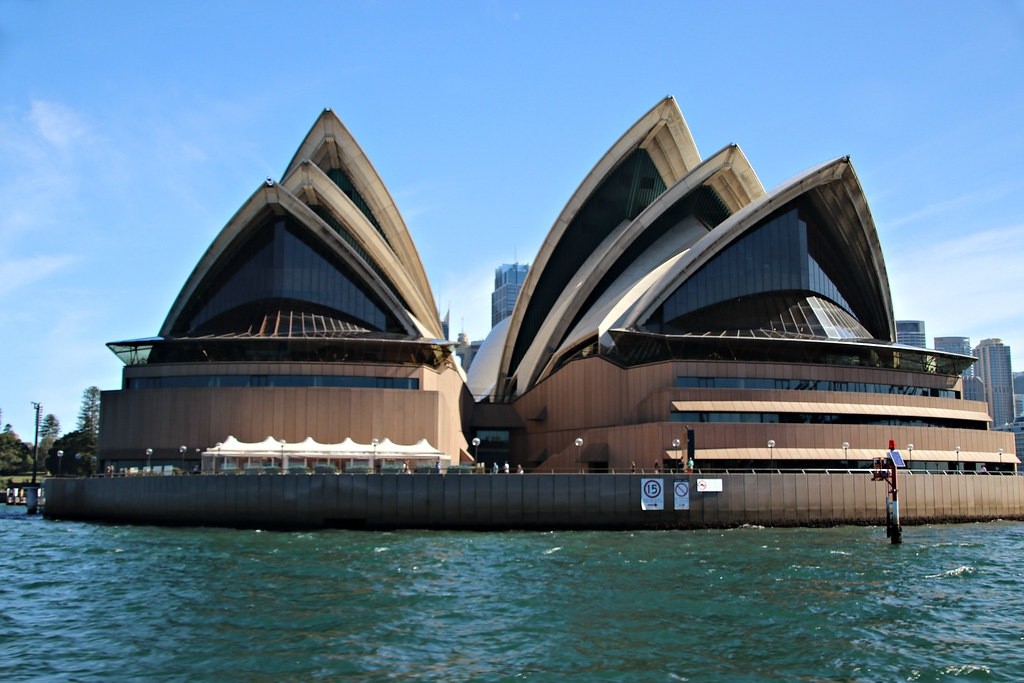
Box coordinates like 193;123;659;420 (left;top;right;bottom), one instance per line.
435;460;442;474
402;459;411;474
492;462;498;473
108;465;114;478
981;465;986;475
654;459;660;473
504;461;509;473
517;464;524;474
687;457;694;473
678;456;684;473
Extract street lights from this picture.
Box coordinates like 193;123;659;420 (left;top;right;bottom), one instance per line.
145;448;153;471
178;445;186;473
842;441;849;468
279;437;286;474
672;439;681;474
955;446;961;470
575;437;584;473
58;449;63;477
371;437;377;473
473;438;480;474
907;442;913;468
998;448;1004;472
767;439;776;468
